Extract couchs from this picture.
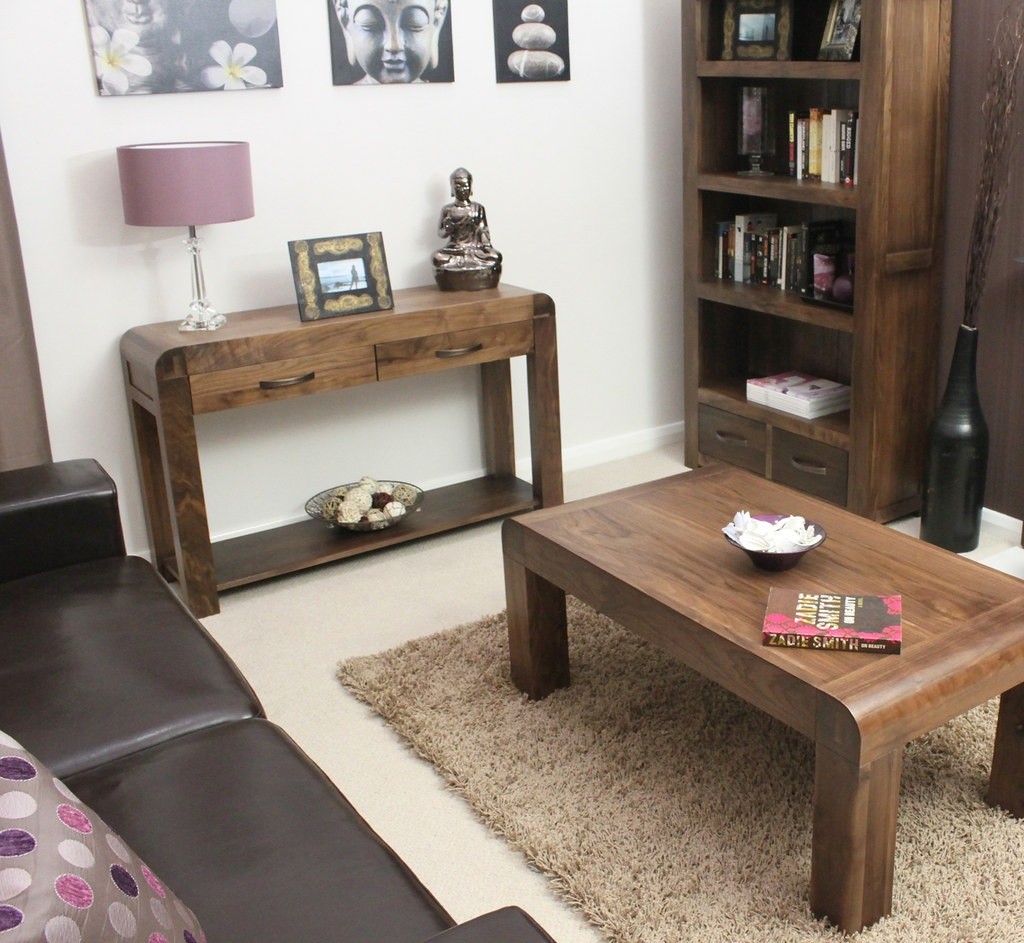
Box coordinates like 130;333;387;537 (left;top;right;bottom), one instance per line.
0;457;558;943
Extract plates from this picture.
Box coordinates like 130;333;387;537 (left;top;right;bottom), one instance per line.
304;479;425;532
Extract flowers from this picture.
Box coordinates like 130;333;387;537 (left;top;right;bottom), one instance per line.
87;24;154;96
198;39;268;93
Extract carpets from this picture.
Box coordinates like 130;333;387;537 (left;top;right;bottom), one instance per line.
334;595;1024;943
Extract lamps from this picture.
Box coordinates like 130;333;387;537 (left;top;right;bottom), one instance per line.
114;139;258;332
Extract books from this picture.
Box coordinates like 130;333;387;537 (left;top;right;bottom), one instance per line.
745;368;852;420
712;212;810;293
784;107;859;185
760;585;906;656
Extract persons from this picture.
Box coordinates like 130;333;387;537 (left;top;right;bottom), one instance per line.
430;166;503;274
347;264;359;291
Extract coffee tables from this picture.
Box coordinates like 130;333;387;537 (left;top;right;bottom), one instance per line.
500;460;1024;940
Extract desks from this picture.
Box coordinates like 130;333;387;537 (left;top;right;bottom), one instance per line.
118;278;566;620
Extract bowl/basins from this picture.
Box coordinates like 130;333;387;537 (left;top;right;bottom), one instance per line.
724;514;827;573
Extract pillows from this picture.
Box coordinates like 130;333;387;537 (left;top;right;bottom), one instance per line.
0;727;209;943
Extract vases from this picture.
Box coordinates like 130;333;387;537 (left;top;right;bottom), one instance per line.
915;321;991;555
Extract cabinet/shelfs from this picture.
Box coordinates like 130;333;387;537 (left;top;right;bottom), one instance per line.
681;0;954;521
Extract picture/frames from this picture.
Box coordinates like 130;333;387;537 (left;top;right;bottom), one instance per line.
722;0;794;61
816;1;863;62
285;231;394;323
